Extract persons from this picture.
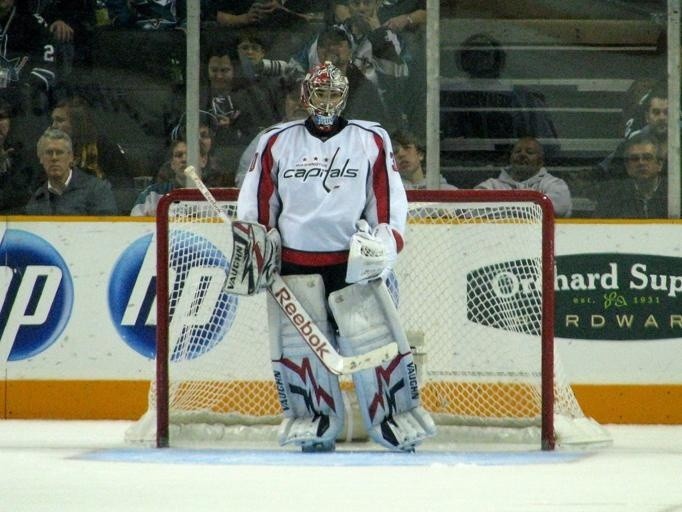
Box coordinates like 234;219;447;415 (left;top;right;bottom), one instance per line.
219;60;440;454
438;32;567;187
471;78;669;216
0;1;424;215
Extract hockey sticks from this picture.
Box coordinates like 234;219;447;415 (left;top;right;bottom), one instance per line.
184;166;399;375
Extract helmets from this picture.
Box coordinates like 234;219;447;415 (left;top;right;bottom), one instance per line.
300;62;349;129
